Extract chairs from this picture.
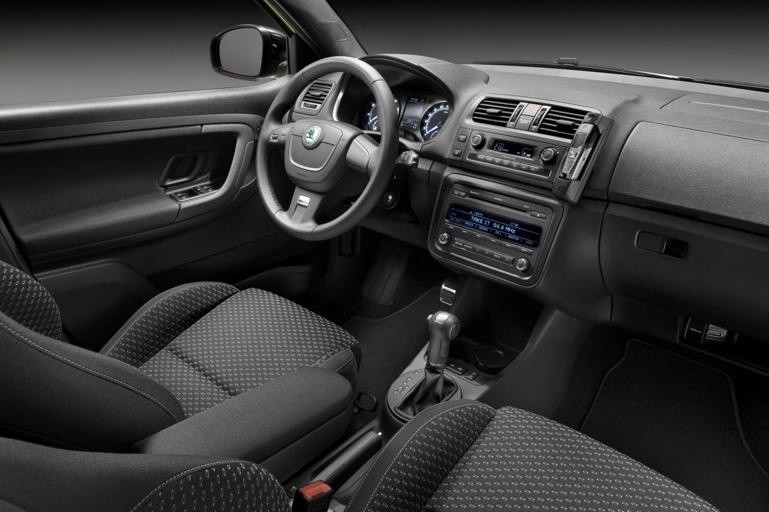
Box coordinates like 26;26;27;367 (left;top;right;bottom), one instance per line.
0;262;362;484
1;399;719;512
